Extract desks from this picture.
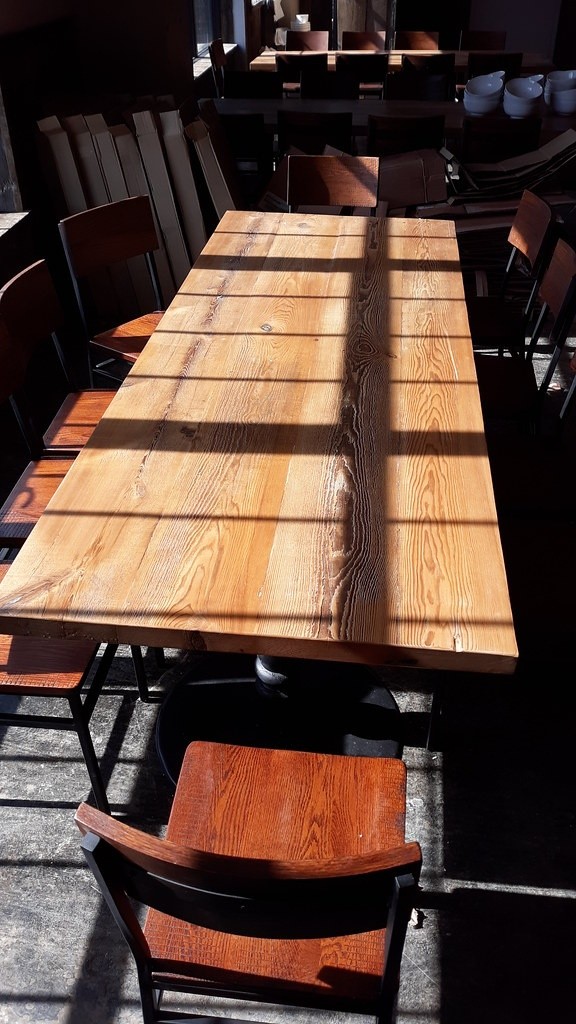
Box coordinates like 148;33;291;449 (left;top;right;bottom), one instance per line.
211;97;575;144
0;211;521;829
247;49;551;81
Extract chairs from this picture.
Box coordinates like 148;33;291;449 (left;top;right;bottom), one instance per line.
202;99;542;165
467;190;576;631
75;740;422;1022
0;196;169;823
287;153;381;215
209;30;522;102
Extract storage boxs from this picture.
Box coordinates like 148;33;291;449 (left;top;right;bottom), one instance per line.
258;145;448;217
36;89;239;322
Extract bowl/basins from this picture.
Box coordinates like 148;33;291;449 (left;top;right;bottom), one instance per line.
463;70;576;120
296;15;308;22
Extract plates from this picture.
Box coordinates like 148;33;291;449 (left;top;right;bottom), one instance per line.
291;22;311;32
